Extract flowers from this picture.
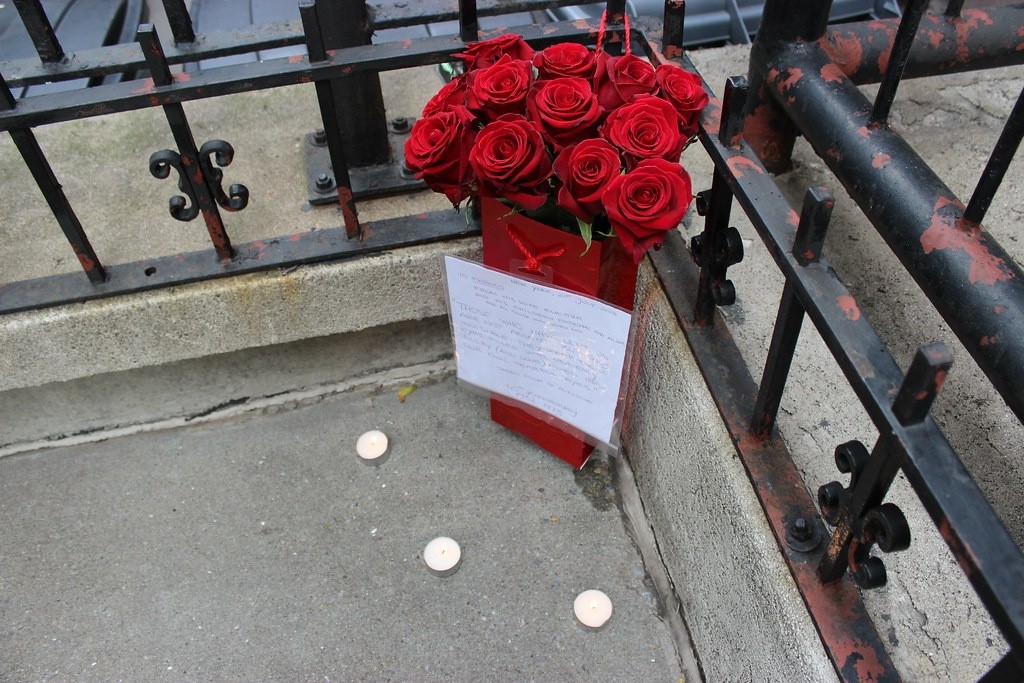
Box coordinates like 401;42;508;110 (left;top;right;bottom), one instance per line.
402;31;710;266
